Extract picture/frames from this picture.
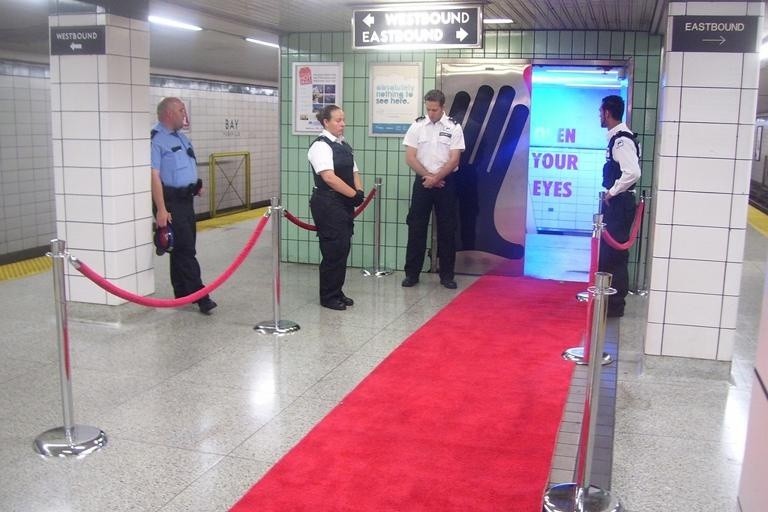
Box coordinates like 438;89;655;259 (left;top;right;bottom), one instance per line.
292;61;343;136
368;61;423;138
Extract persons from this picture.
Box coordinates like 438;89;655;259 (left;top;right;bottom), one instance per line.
599;95;641;317
307;105;364;310
402;89;466;289
151;97;217;312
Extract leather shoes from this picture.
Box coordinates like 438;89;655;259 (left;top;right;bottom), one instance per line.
402;277;419;286
321;300;347;310
198;298;216;312
339;293;353;306
440;279;457;288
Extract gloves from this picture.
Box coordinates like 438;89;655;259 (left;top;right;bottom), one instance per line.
353;190;364;207
